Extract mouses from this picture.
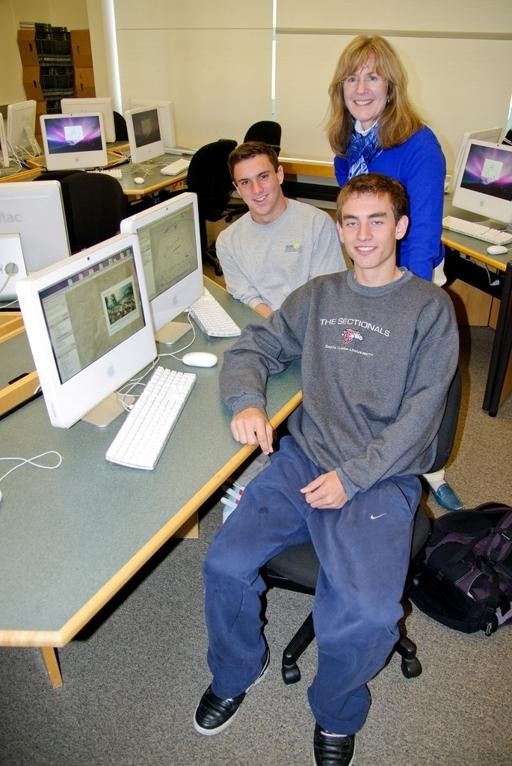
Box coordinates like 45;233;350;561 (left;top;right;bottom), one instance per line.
134;177;144;184
487;246;508;255
182;352;218;367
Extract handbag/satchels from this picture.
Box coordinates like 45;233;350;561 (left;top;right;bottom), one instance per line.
408;502;512;634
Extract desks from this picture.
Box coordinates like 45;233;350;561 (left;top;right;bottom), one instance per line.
433;181;511;418
0;221;315;690
0;127;205;197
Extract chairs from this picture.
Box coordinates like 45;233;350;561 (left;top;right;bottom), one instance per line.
245;363;464;686
223;118;285;225
155;134;239;279
57;171;128;256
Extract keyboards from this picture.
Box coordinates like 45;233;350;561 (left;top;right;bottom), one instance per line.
105;366;197;472
87;169;122;179
160;159;191;176
189;286;241;337
442;216;512;245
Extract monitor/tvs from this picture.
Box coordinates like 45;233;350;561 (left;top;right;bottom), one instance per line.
0;98;176;172
452;126;502;191
120;192;204;344
15;233;158;429
0;180;71;309
451;139;512;230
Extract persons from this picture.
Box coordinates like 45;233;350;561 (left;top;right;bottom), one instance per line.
192;175;460;765
216;144;347;318
318;34;469;512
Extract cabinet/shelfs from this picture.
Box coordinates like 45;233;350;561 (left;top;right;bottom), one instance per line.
18;26;96;130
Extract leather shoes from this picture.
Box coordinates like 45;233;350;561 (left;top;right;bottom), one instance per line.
422;473;463;511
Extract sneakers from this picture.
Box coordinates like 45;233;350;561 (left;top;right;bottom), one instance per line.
315;723;355;765
192;649;271;738
